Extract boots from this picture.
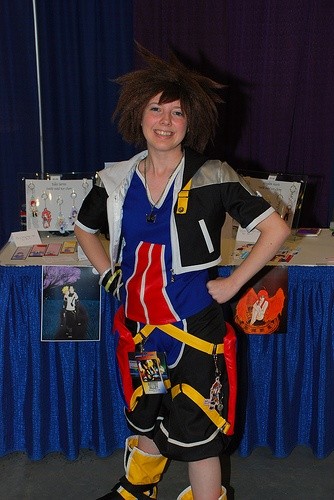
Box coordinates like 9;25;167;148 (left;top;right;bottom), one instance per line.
86;436;167;499
175;482;227;500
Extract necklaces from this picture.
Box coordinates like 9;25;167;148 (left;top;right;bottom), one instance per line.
141;154;186;225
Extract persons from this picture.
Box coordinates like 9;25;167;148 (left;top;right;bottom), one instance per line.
73;61;294;500
63;285;78;337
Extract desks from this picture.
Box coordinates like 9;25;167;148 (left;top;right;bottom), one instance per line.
0;227;334;460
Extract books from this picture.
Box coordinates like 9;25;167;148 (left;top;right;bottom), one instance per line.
296;228;322;236
